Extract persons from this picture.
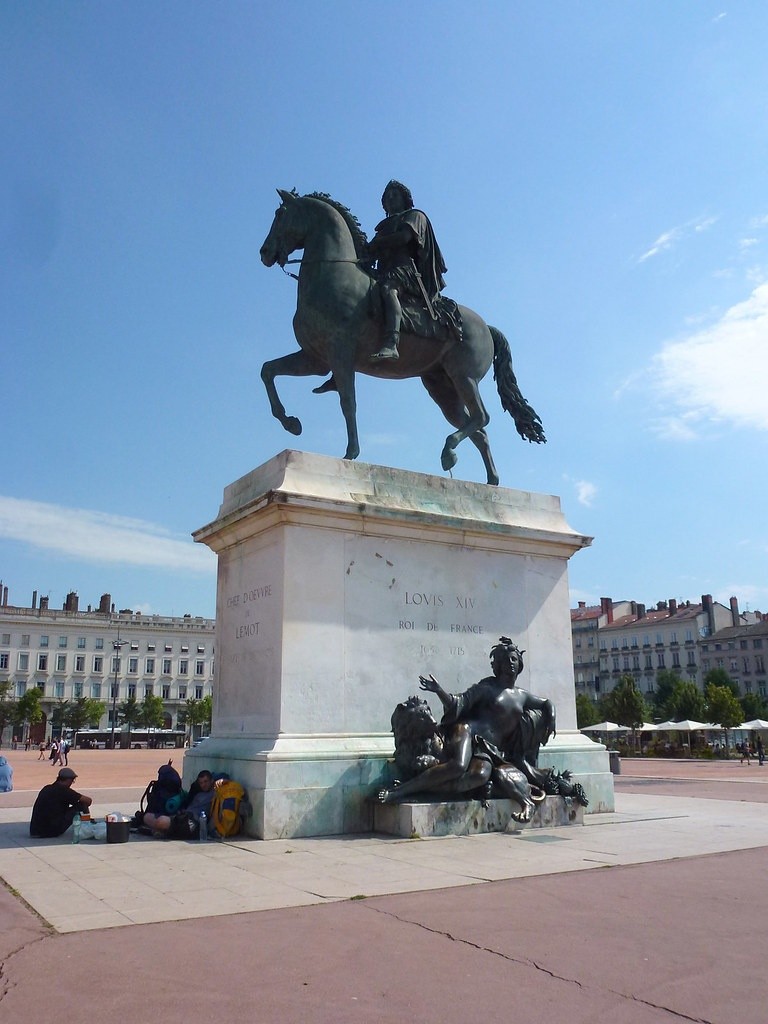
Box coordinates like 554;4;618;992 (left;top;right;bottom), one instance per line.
312;180;433;394
131;770;229;841
379;645;555;801
641;736;766;766
11;735;69;766
30;768;91;837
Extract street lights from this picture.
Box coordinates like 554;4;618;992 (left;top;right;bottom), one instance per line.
110;639;130;748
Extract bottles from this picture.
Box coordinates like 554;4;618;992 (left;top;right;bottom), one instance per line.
199;811;208;843
72;811;82;843
79;815;95;839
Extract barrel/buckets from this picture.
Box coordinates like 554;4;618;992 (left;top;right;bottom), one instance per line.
105;821;129;843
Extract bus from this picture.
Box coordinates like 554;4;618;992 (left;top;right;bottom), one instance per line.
63;728;186;750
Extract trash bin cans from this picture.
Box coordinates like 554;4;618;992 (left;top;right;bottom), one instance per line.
608;751;621;775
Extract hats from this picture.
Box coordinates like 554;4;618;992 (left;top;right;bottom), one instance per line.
57;768;78;779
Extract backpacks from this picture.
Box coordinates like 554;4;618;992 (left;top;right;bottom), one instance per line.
140;780;175;815
65;741;71;753
209;780;249;840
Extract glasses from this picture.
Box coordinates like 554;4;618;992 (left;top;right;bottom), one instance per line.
71;779;76;784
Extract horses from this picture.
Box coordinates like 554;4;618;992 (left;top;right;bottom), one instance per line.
259;187;548;490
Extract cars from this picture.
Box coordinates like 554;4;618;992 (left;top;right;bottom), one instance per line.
192;737;206;747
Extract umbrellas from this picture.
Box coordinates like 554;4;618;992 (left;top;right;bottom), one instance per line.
579;718;768;759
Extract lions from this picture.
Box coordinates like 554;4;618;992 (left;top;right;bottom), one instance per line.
364;696;590;823
